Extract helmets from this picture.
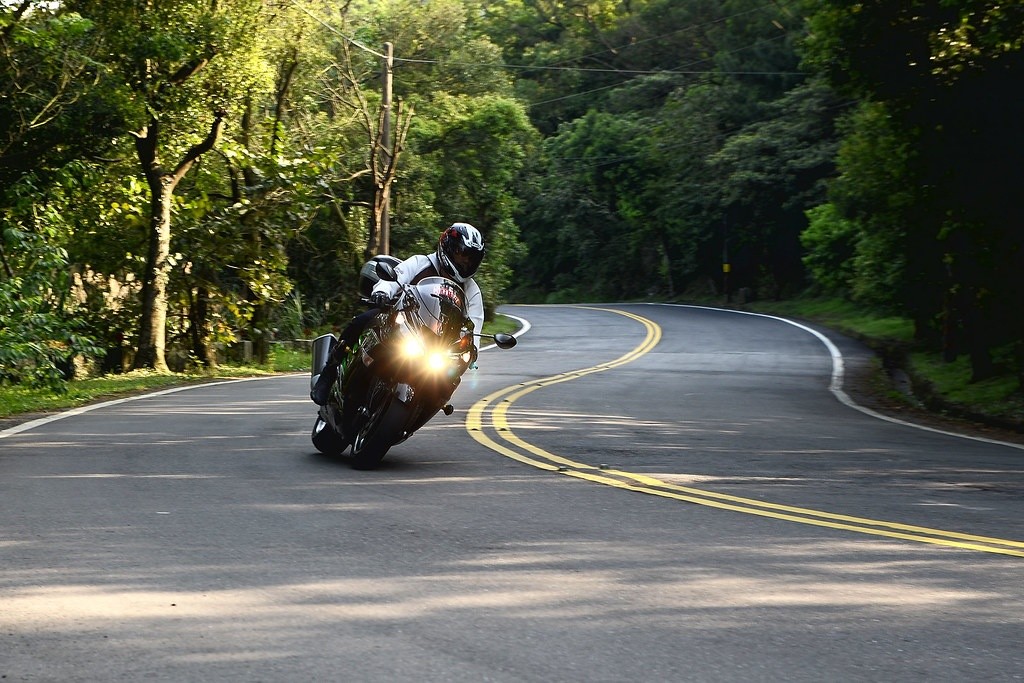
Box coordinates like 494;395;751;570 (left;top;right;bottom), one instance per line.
436;223;485;286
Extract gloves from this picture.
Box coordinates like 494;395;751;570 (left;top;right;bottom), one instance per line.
472;352;477;362
371;291;389;309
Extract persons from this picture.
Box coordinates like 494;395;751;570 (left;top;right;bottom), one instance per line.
310;223;485;406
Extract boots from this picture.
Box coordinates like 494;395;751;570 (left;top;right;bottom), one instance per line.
310;340;348;406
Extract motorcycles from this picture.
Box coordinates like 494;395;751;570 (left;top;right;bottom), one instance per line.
310;253;517;472
